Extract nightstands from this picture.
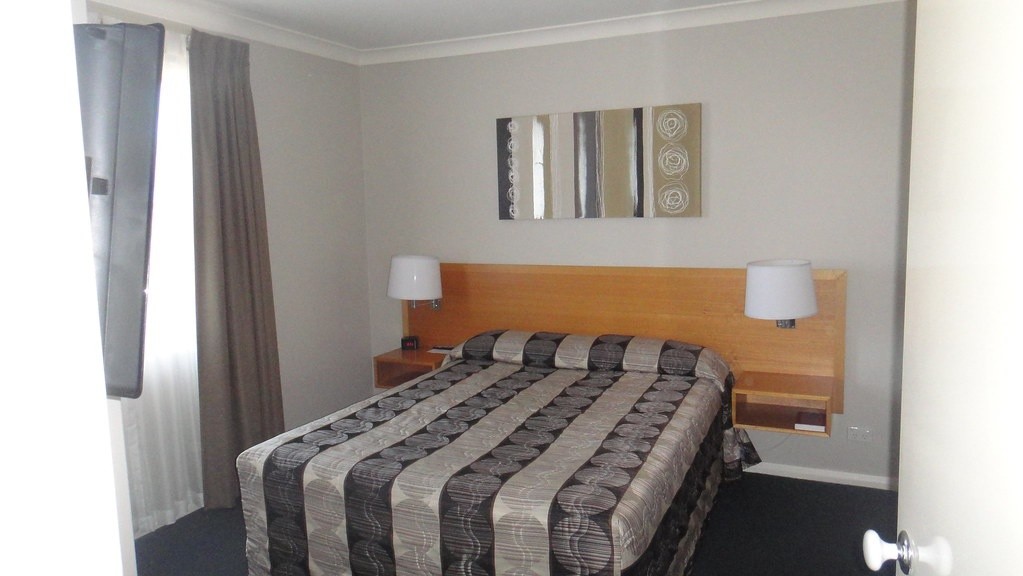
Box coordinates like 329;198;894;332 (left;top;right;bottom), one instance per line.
731;371;836;438
374;347;444;387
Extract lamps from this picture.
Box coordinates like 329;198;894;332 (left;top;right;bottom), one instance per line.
742;258;819;330
386;254;442;312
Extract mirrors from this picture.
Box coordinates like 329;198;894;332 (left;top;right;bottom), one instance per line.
496;102;703;221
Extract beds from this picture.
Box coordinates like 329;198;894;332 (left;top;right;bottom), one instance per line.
236;261;846;576
